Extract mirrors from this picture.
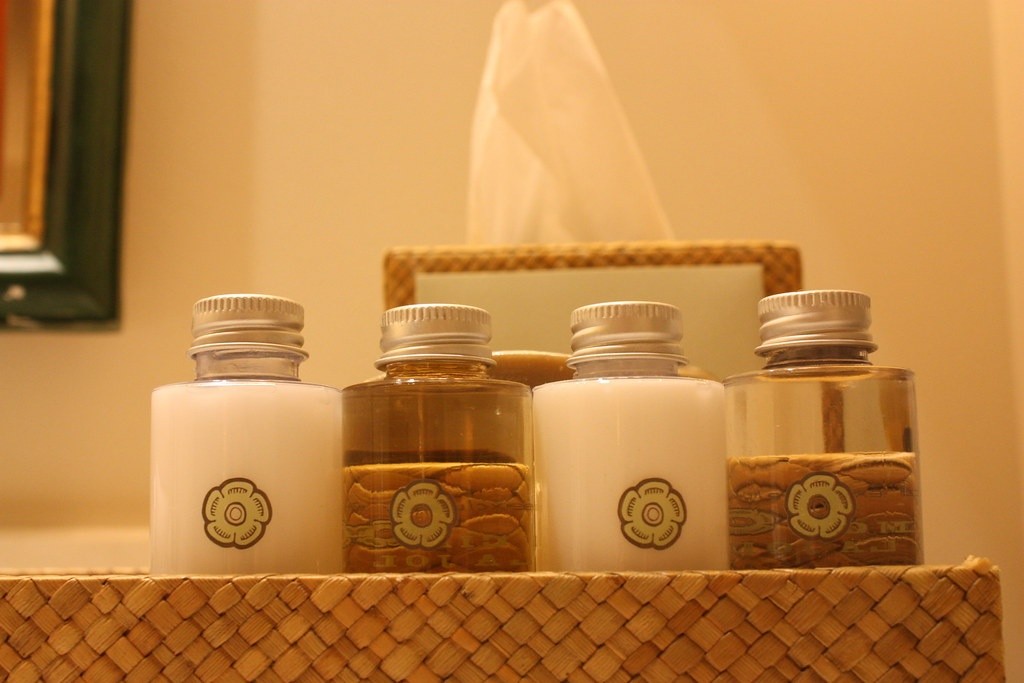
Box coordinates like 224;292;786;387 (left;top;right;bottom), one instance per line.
0;0;132;339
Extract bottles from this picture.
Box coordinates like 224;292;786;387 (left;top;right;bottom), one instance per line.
536;302;724;572
343;304;533;574
149;294;343;575
724;288;927;569
